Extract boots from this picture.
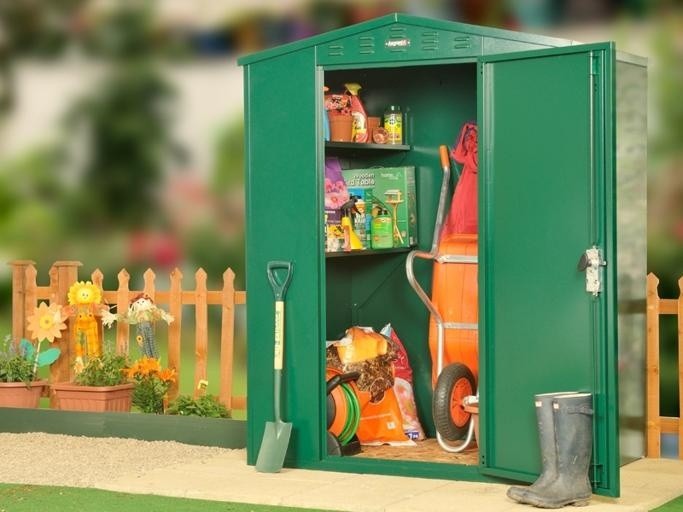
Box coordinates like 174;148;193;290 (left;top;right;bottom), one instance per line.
522;392;594;510
506;391;579;504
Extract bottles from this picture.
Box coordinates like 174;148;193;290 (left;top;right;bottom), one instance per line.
370;209;393;250
384;105;403;145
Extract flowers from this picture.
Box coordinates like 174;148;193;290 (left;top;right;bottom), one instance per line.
119;354;233;419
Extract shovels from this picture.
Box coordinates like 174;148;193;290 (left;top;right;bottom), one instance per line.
256;260;292;472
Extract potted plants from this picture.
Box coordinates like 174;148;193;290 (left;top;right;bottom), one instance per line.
0;332;136;412
